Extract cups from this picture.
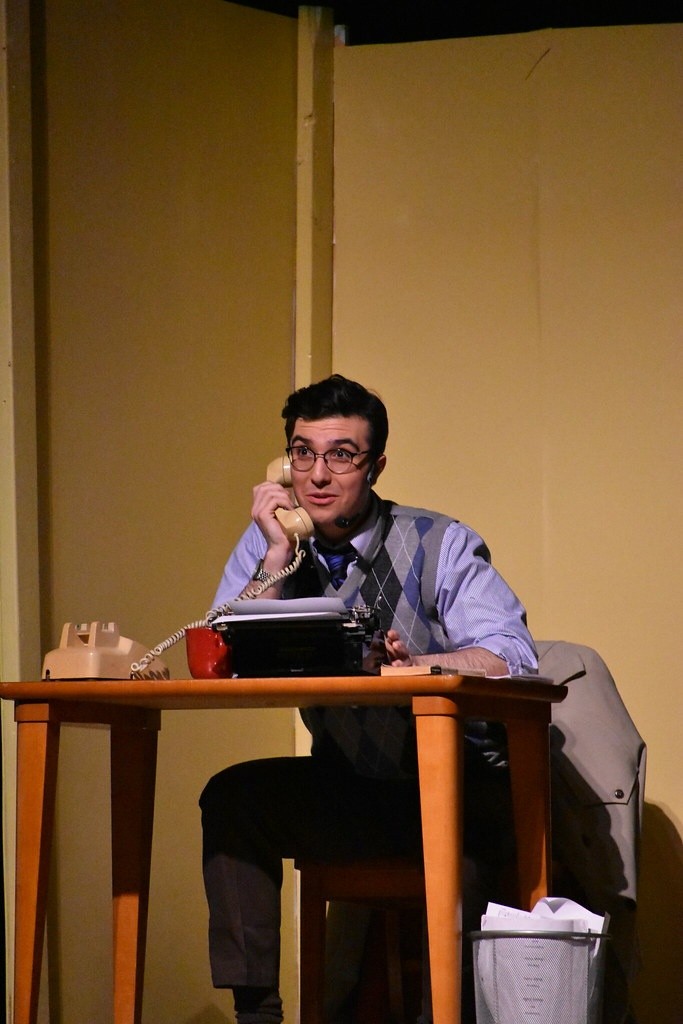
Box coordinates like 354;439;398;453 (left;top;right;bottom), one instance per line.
185;623;236;679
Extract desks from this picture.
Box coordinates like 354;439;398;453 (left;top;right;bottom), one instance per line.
0;672;568;1024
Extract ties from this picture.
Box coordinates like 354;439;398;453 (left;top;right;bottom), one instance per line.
327;546;358;590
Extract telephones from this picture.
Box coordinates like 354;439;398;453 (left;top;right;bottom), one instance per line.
266;455;316;541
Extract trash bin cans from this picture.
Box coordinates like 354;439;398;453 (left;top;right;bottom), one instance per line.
469;931;614;1024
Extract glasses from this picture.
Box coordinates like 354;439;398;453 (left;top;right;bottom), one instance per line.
286;442;372;474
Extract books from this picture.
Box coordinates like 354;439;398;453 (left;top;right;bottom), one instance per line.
380;663;486;678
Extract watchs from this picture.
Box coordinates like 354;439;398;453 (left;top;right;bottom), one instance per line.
252;558;283;590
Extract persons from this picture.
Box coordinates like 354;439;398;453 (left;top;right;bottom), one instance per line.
199;375;639;1023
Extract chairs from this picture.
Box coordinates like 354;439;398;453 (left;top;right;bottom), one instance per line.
295;642;607;1024
